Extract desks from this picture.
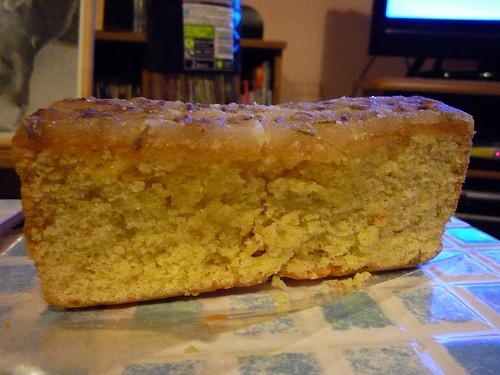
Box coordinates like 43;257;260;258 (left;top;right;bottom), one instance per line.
2;197;500;374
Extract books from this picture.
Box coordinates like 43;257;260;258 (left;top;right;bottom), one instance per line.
95;59;272;107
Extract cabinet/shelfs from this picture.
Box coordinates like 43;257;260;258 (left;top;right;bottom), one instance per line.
76;2;283;108
366;77;498;229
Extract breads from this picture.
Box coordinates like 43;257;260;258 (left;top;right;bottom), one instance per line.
11;92;476;310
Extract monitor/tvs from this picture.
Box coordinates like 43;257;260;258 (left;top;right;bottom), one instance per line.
368;0;499;59
